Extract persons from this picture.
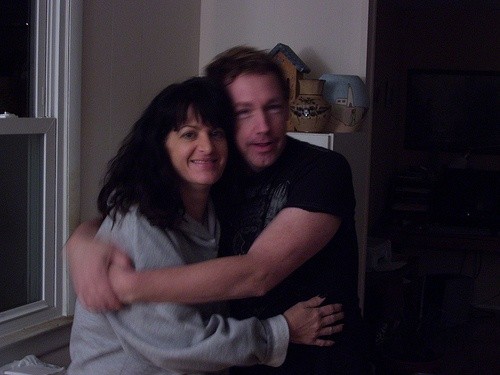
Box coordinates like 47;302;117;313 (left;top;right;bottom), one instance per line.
69;49;363;375
69;77;343;375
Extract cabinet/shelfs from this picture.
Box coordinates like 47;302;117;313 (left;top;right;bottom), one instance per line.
285;131;365;307
